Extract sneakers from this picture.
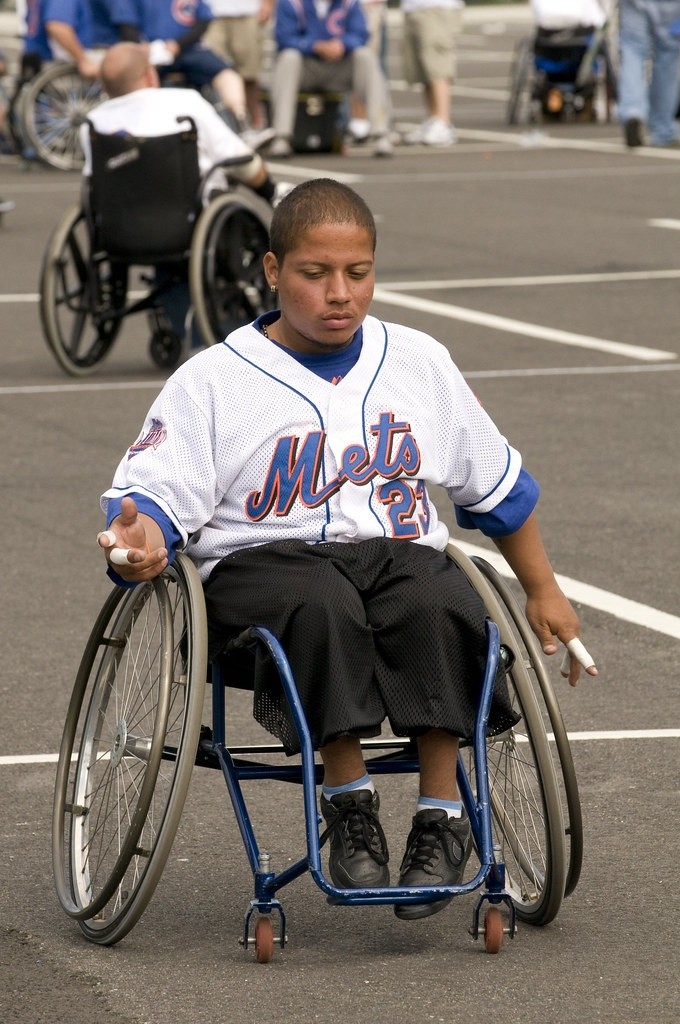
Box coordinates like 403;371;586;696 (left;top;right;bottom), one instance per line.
320;788;390;889
394;804;474;920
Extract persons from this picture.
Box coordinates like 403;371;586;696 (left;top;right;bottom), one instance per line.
97;177;597;920
69;42;278;373
19;0;680;178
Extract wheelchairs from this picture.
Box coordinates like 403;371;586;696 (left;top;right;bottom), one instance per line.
6;52;112;173
52;541;583;964
39;118;275;378
503;24;617;129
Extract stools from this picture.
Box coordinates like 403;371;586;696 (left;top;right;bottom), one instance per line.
256;91;348;154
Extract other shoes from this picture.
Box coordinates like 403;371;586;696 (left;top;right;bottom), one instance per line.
404;118;457;148
269;139;292;158
370;136;393;156
624;118;644;147
